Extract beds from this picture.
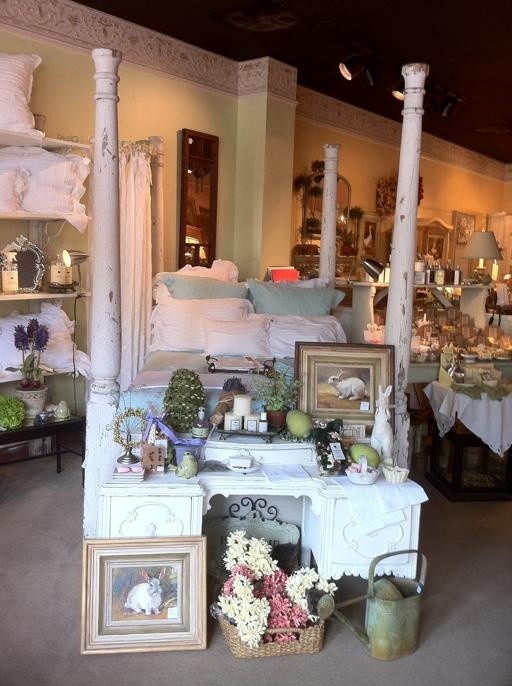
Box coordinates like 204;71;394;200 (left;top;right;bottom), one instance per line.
83;46;432;536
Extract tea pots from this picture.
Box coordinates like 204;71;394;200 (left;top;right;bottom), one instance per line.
55;400;69;418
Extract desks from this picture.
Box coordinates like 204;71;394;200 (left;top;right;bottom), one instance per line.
0;413;86;487
351;280;497;343
97;428;420;581
424;379;512;502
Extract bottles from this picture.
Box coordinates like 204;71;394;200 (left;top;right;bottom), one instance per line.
48;252;74;286
258;412;269;432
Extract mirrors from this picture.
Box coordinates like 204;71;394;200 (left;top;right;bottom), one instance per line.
1;234;47;293
180;127;219;268
301;170;352;239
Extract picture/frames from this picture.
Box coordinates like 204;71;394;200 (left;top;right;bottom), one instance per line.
293;340;397;436
355;209;383;266
186;196;200;227
79;535;208;655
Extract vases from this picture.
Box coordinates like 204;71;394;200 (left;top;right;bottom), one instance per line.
15;387;49;422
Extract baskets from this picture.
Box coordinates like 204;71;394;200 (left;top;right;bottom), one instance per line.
213;602;324;659
382;466;410;483
345;455;378;485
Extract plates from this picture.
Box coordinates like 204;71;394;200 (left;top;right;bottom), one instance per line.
225;461;263;474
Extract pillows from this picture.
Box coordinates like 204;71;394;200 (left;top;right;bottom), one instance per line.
0;300;92;379
145;259;346;362
0;51;92;233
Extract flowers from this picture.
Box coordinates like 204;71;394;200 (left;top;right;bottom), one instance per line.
3;316;56;390
216;528;339;649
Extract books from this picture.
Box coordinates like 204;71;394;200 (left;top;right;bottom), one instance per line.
270;269;300;283
205;353;270;374
264;265;295;281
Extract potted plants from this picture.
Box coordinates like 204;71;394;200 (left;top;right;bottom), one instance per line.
339;227;359;255
247;363;305;431
161;368;206;438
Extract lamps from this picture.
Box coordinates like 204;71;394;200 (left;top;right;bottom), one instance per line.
457;231;504;281
344;256;384;288
62;246;90;297
